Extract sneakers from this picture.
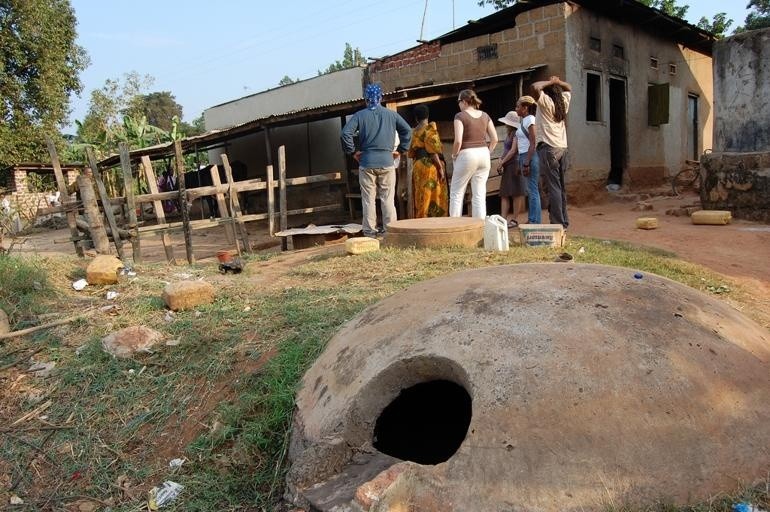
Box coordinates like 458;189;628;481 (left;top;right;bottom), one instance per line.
508;219;517;228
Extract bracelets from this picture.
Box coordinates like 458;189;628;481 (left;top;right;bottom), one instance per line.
451;153;457;159
523;164;531;168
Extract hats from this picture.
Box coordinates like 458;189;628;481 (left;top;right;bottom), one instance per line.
498;111;520;129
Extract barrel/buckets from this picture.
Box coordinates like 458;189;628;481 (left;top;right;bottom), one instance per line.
691;210;731;225
484;214;510;253
637;218;658;230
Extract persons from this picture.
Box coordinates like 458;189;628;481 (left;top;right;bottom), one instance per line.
449;88;499;223
529;76;574;234
62;167;103;215
408;105;450;218
156;170;180;215
514;93;544;224
495;109;525;228
340;82;412;238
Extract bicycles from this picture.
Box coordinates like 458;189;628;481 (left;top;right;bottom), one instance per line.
672;149;712;197
